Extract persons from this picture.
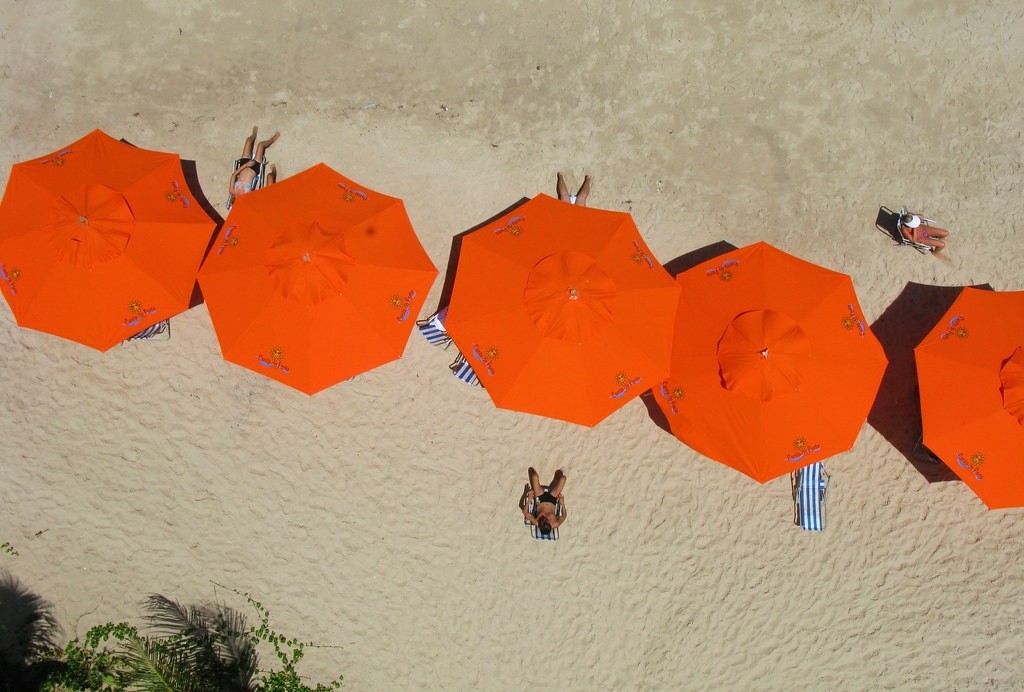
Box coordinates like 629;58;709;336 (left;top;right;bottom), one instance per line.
557;173;590;207
901;214;949;260
523;472;567;534
228;125;281;204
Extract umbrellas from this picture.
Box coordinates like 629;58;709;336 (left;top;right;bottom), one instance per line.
914;286;1024;510
443;193;682;427
645;241;891;483
0;129;218;351
188;163;438;395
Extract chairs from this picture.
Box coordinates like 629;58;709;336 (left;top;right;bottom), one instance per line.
523;488;562;541
127;318;170;341
897;205;937;255
226;152;267;210
794;461;832;532
416;305;453;350
453;354;480;387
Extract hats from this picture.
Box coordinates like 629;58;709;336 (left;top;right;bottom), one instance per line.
902;215;921;228
